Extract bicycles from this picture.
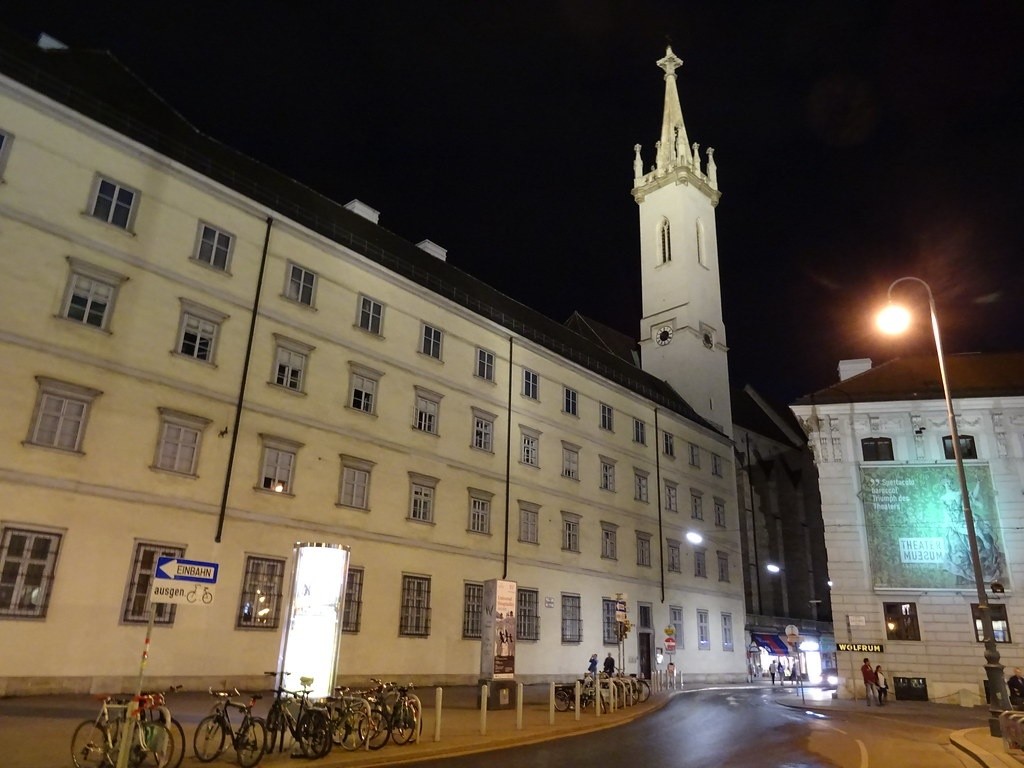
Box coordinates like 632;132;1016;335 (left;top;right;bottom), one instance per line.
263;671;422;758
193;686;268;767
592;666;651;708
71;683;186;768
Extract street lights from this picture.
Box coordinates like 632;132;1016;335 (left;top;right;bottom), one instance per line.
874;277;1010;738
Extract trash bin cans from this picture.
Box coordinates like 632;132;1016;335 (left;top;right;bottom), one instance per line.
893;676;929;701
477;679;517;710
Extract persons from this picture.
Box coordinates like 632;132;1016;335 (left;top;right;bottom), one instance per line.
769;660;786;685
1008;668;1024;712
874;665;889;705
588;653;599;677
789;663;799;685
861;657;878;706
604;653;614;678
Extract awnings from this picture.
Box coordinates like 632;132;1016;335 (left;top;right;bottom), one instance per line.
754;632;789;656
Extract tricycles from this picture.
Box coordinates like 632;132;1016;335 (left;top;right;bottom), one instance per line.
554;678;607;713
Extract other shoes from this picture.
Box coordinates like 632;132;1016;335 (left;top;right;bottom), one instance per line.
867;705;871;706
876;704;879;706
